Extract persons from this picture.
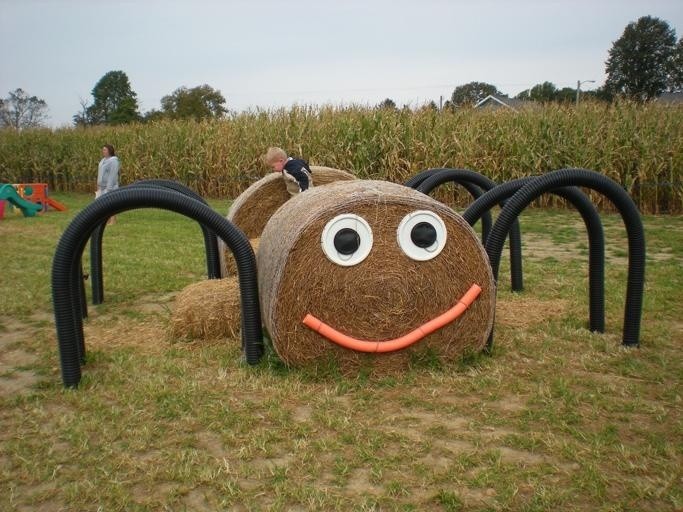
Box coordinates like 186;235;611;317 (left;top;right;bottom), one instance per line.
96;144;120;226
265;147;314;198
